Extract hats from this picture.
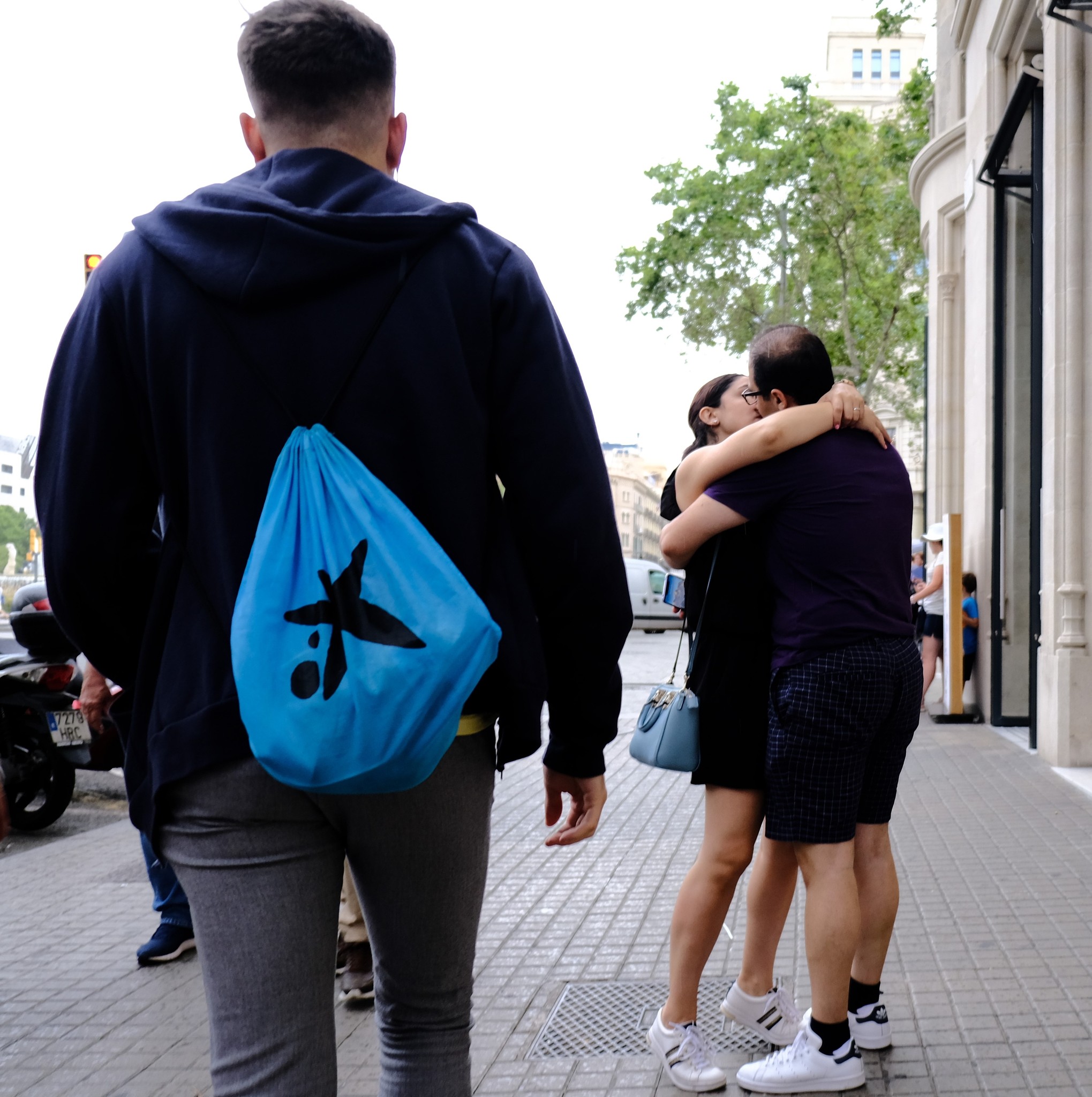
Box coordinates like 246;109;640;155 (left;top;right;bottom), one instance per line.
920;523;944;543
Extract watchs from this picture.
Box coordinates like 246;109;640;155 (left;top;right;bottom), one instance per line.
832;379;855;389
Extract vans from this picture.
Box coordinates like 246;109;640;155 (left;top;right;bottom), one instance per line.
623;556;690;635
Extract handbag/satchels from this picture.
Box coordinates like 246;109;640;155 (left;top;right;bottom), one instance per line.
629;673;701;772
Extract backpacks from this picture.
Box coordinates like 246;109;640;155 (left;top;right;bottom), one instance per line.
227;419;504;798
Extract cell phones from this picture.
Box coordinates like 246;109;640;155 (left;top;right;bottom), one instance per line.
662;572;686;609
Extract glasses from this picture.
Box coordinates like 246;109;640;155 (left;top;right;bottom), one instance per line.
741;388;771;406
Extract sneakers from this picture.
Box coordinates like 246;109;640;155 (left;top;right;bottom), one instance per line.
136;922;196;964
720;974;803;1046
647;1006;728;1092
335;928;375;1002
735;1015;867;1093
802;993;892;1050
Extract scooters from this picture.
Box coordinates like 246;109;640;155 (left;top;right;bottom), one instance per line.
0;580;124;832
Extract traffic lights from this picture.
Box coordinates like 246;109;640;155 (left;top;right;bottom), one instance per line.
84;254;102;286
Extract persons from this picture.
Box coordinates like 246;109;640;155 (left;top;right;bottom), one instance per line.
75;647;195;967
336;851;385;1011
645;374;893;1090
658;322;925;1092
936;571;980;703
33;2;631;1097
911;537;927;649
910;523;944;708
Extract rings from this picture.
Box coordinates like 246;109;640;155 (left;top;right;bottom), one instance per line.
853;407;860;412
